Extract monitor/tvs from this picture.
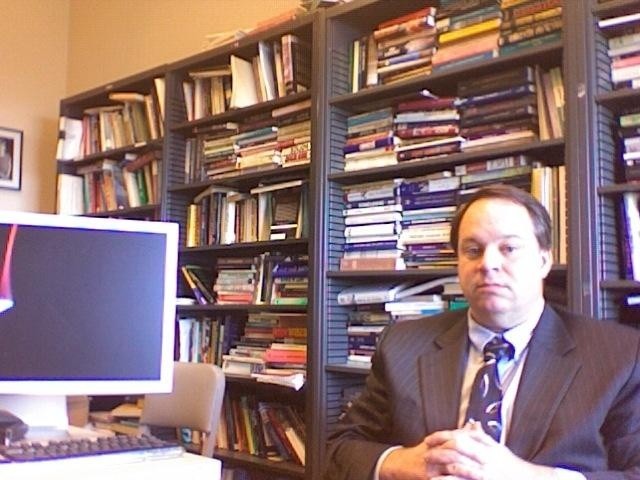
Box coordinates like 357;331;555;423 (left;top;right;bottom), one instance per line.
0;209;179;441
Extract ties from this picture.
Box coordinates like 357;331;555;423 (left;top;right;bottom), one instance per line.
461;334;516;448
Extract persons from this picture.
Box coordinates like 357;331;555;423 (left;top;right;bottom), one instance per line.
320;182;640;479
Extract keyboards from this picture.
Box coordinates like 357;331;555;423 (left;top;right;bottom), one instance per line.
0;433;184;470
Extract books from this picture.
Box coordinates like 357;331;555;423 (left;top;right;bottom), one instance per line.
336;0;565;367
86;393;320;479
55;77;166;215
598;12;640;305
181;3;307;390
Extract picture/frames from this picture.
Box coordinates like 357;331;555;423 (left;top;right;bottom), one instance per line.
0;124;25;191
